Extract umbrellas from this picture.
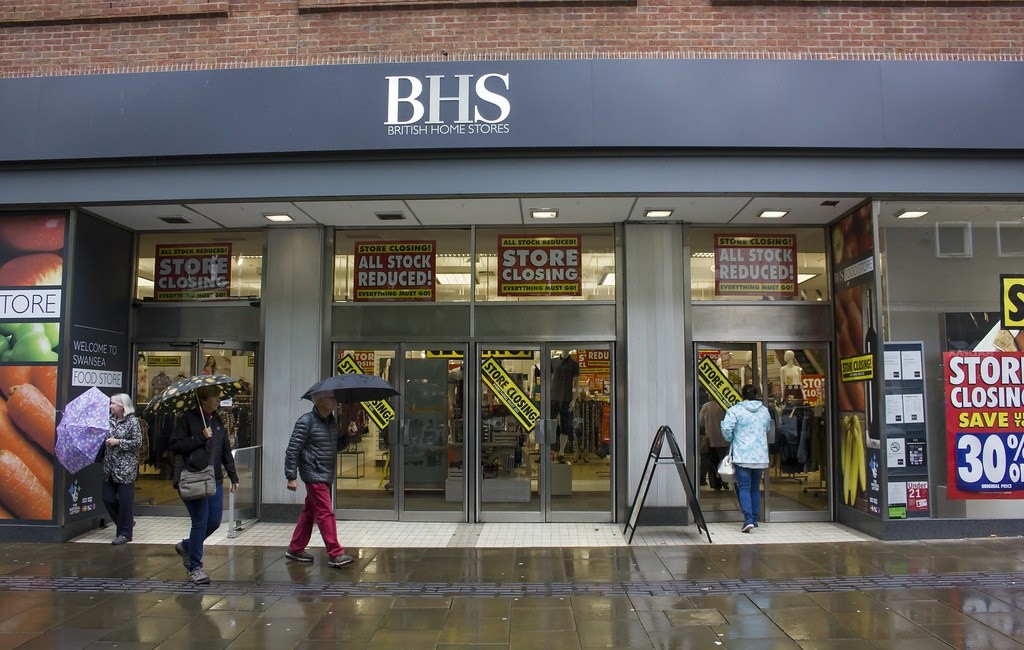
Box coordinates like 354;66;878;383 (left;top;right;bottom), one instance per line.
300;371;401;421
55;386;110;474
144;375;250;429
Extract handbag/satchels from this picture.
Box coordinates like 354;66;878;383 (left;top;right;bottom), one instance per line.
717;443;735;476
178;463;218;499
699;435;710;454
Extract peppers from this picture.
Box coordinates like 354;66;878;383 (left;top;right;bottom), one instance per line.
0;322;60;361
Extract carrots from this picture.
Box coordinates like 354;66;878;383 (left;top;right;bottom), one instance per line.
834;284;865;411
0;366;58;521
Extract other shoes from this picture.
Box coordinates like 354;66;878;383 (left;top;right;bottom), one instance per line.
700;481;708;485
723;482;729;490
714;487;720;491
111;535;132;546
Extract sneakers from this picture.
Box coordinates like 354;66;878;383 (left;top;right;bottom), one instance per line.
328;553;354;568
176;540;196;572
187;566;212;584
740;519;754;532
284;546;313;561
754;522;759;530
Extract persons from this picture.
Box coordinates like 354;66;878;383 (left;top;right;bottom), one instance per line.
282;388;357;565
100;393;142;543
776;350;809;402
203;357;217;375
170;385;240;582
548;350;580;462
699;391;730;492
723;384;774;533
528;361;541;451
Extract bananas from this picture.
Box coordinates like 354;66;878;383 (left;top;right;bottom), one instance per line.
839;414;866;506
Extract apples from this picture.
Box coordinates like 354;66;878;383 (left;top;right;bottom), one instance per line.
0;213;65;286
